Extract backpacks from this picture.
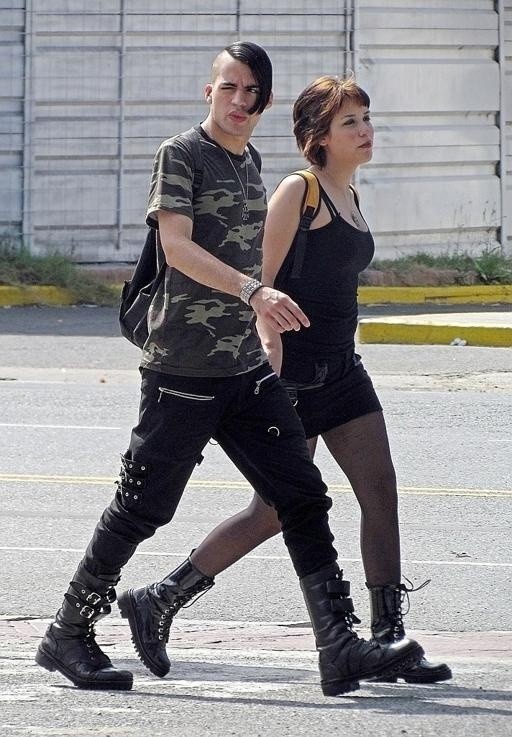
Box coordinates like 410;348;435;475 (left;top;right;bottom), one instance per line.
119;130;204;349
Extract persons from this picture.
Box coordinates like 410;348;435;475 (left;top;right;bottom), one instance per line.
117;74;453;686
37;38;424;697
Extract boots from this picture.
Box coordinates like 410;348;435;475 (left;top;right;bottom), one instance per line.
299;562;453;697
35;548;134;690
117;548;215;678
